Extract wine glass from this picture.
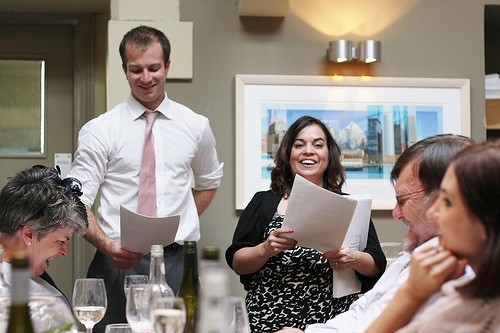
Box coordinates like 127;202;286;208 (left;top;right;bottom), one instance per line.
72;279;108;333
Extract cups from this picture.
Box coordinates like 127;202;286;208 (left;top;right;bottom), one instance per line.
151;297;186;333
125;285;165;333
105;324;132;333
124;275;148;300
227;296;250;333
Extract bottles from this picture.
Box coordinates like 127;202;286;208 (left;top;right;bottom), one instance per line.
178;241;200;333
149;244;175;297
5;252;37;333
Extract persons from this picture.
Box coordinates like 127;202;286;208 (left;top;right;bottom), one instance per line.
364;136;500;333
279;133;474;333
65;24;224;333
226;115;387;333
0;165;89;333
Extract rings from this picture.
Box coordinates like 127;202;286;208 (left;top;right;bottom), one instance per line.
334;263;338;268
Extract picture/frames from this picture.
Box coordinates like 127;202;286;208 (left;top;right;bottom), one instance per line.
234;74;470;211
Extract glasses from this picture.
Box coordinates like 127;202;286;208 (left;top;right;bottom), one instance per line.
395;186;431;207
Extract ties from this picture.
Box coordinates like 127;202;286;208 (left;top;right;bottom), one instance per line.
138;111;160;217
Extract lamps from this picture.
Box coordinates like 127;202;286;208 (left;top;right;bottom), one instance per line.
326;40;381;62
